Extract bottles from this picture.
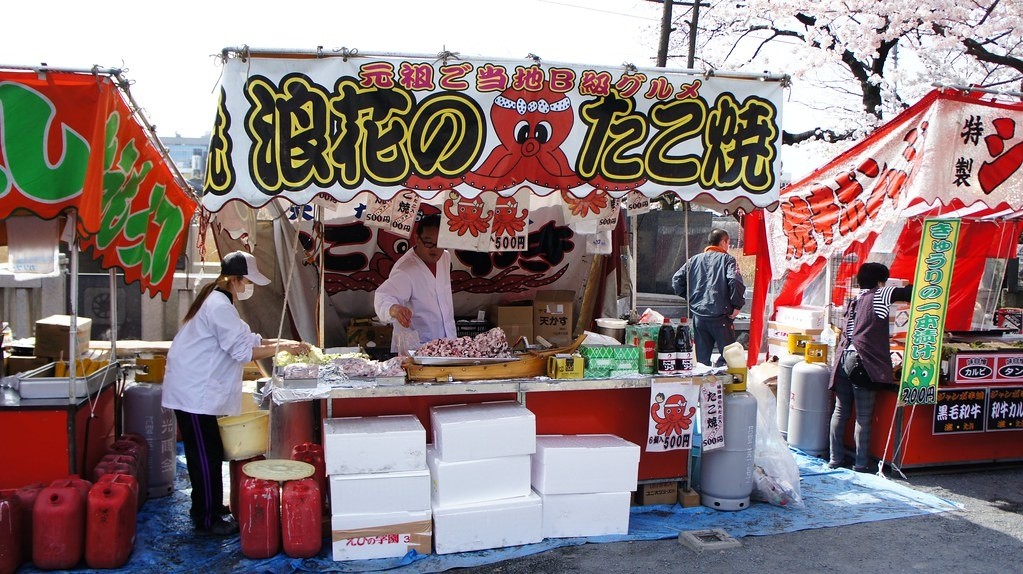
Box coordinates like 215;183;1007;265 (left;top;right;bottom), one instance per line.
656;318;676;375
639;333;655;373
628;330;639;346
676;317;693;373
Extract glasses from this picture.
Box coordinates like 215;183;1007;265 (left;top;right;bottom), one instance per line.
418;235;436;249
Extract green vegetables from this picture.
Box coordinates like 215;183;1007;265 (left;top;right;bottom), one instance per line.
1010;340;1023;348
942;345;957;358
968;340;988;348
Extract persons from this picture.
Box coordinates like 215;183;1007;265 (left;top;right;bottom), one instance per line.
160;250;310;537
672;228;746;366
828;262;913;471
374;214;458;359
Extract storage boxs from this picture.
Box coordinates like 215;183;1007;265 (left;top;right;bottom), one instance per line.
323;401;678;562
768;306;825;359
454;290;695;379
347;324;393;350
8;314;92;375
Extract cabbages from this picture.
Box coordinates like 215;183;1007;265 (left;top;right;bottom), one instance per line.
275;345;365;366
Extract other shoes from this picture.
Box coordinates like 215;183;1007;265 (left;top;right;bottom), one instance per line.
219;504;234;518
197;515;238;537
852;462;878;471
829;455;854;468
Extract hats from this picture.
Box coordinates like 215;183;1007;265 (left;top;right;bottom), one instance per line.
221;250;272;285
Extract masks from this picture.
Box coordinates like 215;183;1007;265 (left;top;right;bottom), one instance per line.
229;275;255;300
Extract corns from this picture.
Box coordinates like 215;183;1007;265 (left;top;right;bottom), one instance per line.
55;358;109;378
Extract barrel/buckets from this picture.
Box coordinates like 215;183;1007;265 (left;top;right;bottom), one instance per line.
595;326;626;344
216;409;325;556
0;433;150;572
